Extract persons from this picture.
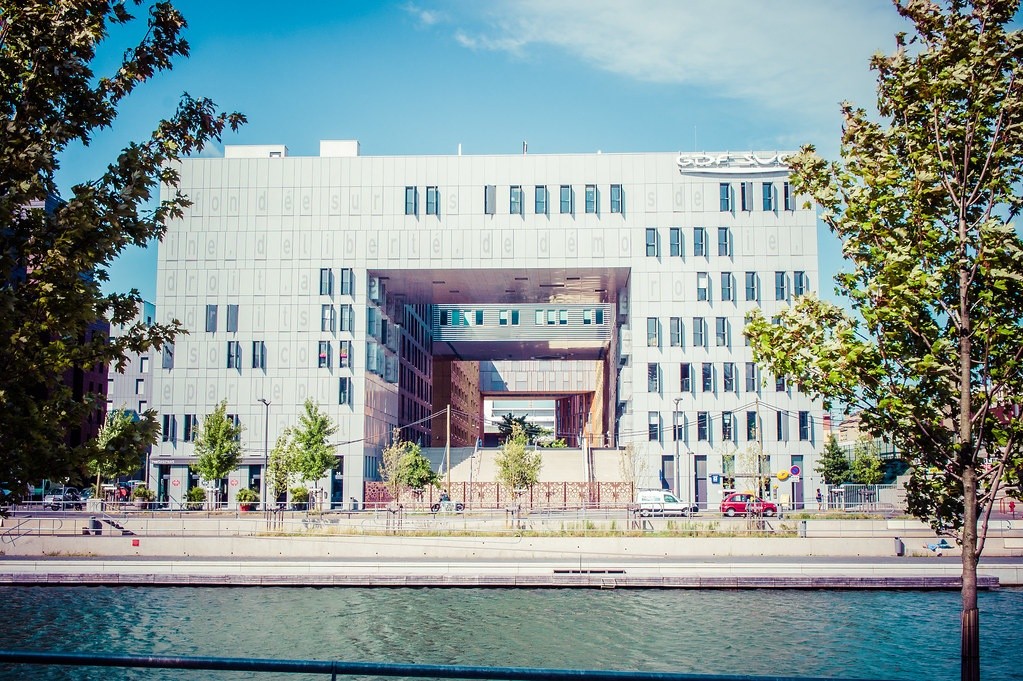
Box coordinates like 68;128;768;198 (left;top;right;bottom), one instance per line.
923;539;954;557
816;489;823;510
71;492;81;507
479;438;482;447
440;489;449;511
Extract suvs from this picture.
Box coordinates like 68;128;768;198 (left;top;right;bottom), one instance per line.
637;491;699;517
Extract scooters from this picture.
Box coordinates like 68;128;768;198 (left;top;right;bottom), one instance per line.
431;495;464;513
51;493;83;511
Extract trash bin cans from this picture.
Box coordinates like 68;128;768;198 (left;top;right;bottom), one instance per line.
777;503;783;520
799;521;806;538
894;536;902;556
350;497;354;510
89;516;96;532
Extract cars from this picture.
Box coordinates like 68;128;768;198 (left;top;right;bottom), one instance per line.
43;487;81;508
720;493;777;517
77;479;143;506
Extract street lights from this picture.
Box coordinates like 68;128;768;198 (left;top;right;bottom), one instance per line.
687;449;695;517
257;398;269;511
674;397;685;499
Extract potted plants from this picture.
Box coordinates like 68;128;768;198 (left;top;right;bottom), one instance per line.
237;490;257;510
132;488;148;505
292;488;310;509
184;487;205;510
